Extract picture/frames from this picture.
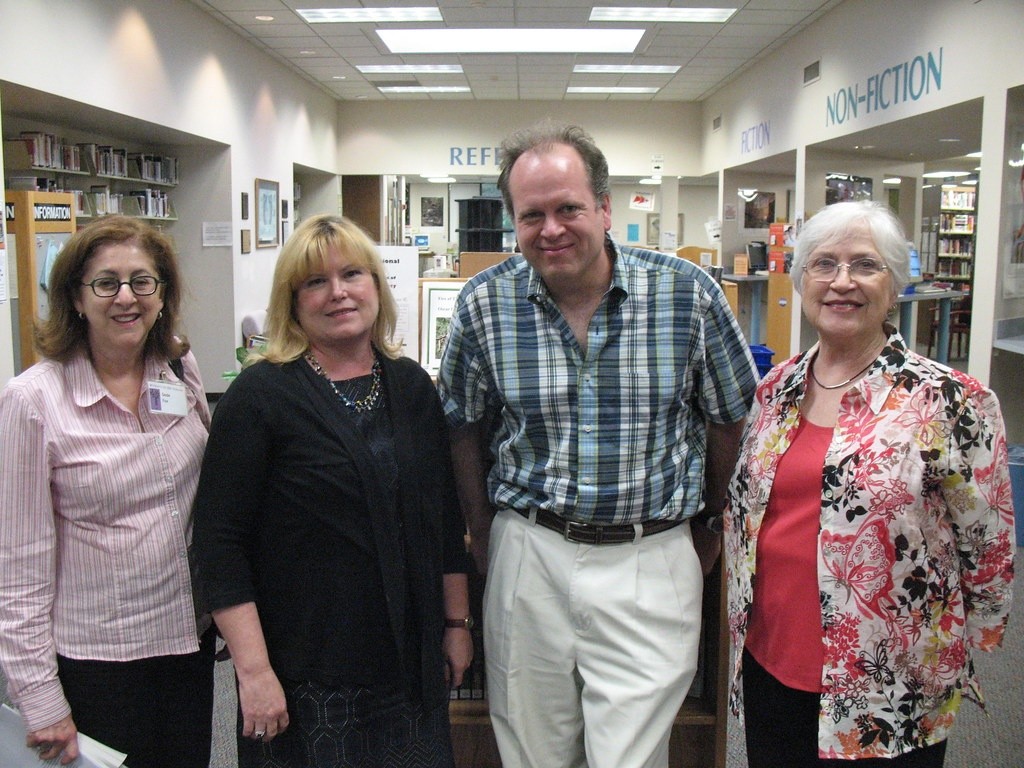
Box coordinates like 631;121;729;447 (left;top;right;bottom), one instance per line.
255;178;280;248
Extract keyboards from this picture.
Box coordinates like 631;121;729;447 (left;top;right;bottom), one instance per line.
915;286;947;294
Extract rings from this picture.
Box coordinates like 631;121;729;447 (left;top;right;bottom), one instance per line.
255;731;265;738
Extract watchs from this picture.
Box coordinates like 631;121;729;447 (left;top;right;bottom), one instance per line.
446;616;474;630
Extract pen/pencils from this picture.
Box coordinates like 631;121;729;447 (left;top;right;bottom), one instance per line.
159;370;165;380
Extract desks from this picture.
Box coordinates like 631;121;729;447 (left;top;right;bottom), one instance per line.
720;273;769;347
896;291;962;364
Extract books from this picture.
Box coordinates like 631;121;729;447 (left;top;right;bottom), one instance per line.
9;131;179;218
938;215;972;295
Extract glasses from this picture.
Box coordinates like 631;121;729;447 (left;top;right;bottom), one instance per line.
802;259;890;281
81;275;164;297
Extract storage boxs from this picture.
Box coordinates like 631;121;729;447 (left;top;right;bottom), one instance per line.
749;346;775;379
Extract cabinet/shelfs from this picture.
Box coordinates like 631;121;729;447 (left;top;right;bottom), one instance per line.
3;141;178;221
931;185;977;329
452;505;731;768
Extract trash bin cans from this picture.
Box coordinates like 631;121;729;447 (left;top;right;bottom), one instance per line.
1007;443;1024;547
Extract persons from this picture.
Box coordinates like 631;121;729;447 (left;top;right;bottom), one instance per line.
435;126;762;768
724;202;1016;768
0;213;212;768
193;219;474;768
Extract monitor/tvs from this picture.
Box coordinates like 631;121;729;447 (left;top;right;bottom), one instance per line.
908;249;924;283
746;244;767;271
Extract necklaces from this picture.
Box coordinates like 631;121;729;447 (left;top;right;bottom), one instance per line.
811;357;876;389
305;349;381;413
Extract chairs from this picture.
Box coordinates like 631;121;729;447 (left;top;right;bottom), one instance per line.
926;295;973;360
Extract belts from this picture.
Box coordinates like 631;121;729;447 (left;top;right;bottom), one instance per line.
512;504;685;544
